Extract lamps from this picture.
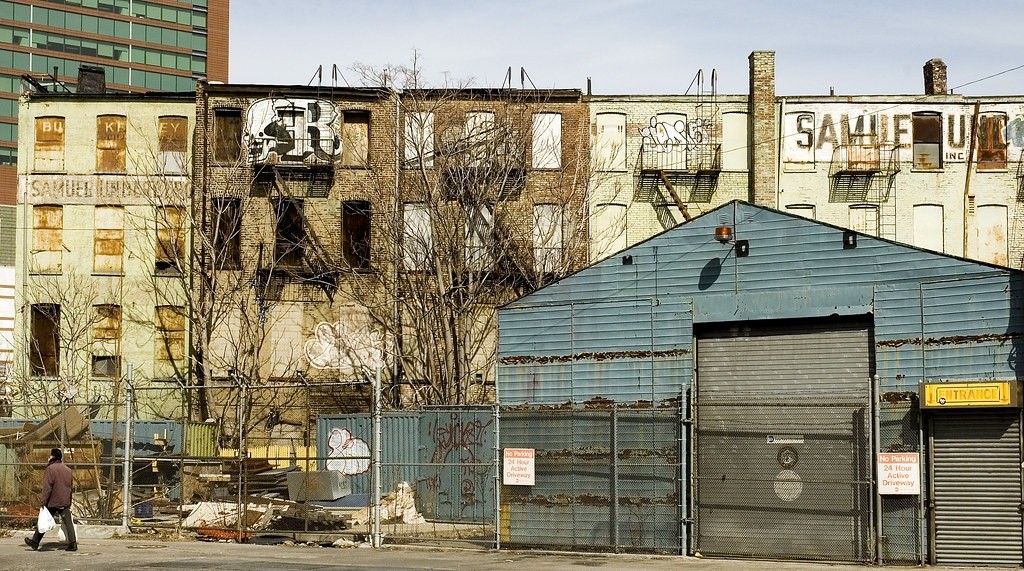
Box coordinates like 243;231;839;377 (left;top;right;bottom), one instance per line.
714;214;733;245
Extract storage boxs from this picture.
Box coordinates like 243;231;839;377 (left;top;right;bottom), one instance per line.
134;501;153;518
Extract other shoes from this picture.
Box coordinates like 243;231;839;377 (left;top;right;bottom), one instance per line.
25;537;38;550
65;543;77;551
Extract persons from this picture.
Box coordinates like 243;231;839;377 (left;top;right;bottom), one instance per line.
24;448;78;552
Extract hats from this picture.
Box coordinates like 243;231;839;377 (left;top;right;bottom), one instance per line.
51;448;62;459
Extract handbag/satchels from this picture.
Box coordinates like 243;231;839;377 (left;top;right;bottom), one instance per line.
37;506;56;533
